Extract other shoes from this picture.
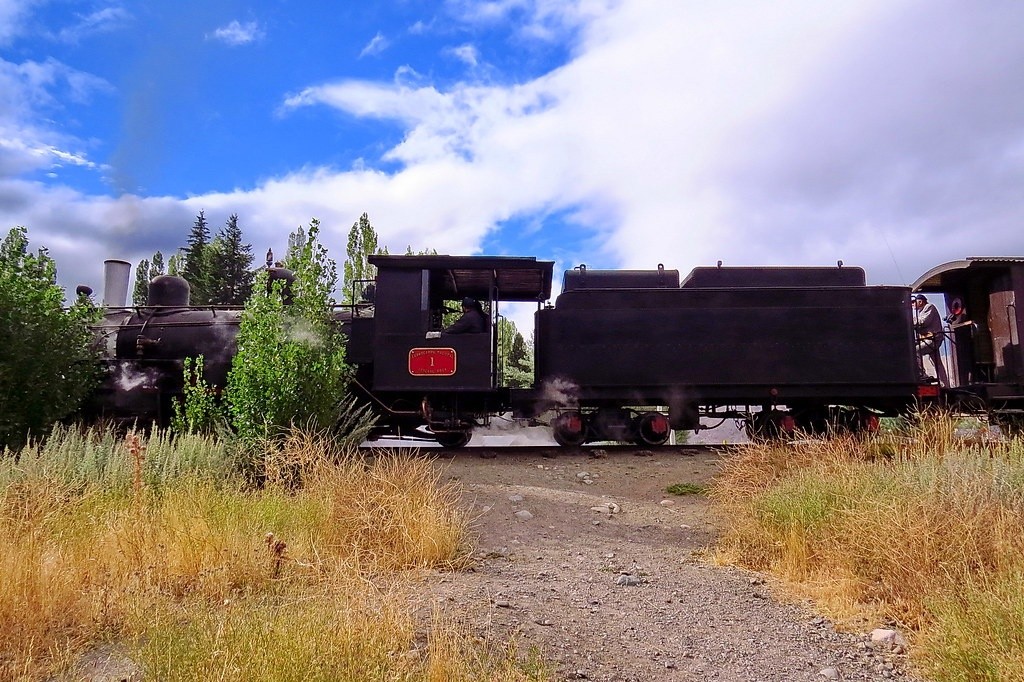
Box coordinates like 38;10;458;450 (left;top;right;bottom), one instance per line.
940;386;950;390
919;375;928;380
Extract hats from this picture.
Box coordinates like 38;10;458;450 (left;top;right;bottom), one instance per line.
916;295;927;300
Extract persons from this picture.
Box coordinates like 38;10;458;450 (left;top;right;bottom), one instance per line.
913;294;949;387
443;296;489;333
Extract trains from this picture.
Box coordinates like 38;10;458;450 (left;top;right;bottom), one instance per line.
65;254;1024;447
